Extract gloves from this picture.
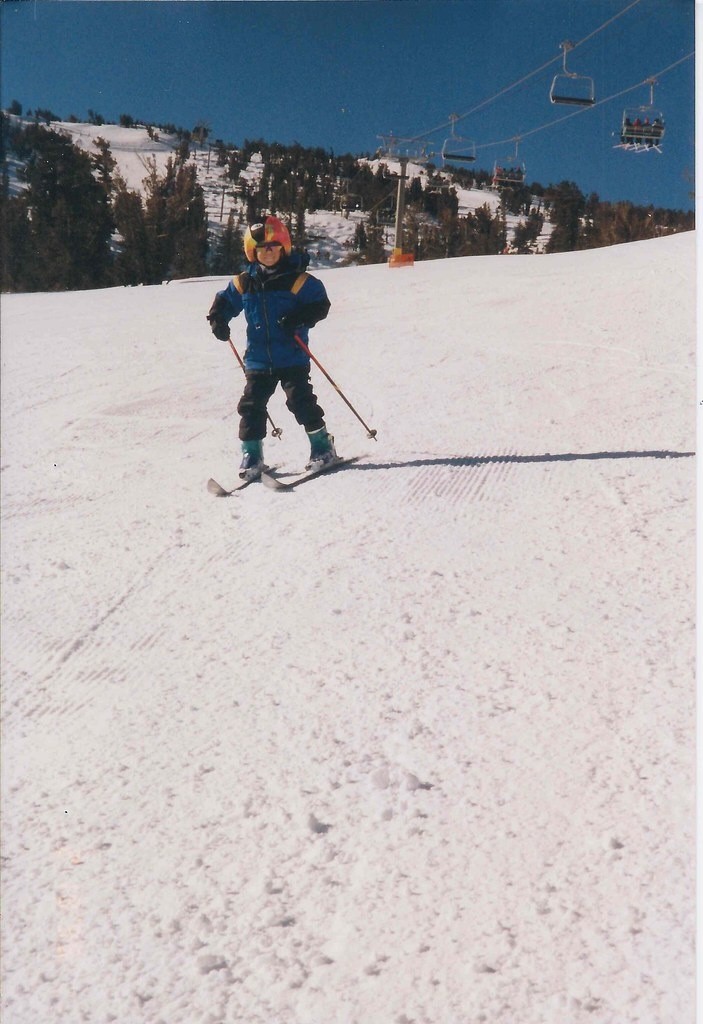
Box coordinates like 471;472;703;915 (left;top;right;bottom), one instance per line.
277;313;304;338
211;318;230;341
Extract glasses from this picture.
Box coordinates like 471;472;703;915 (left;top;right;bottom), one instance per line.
255;246;283;255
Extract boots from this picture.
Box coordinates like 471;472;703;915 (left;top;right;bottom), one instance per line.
304;425;336;469
239;439;263;480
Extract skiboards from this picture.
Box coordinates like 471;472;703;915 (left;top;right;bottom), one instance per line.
202;447;370;496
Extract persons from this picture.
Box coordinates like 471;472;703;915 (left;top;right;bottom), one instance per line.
492;166;523;189
620;117;664;148
208;220;339;476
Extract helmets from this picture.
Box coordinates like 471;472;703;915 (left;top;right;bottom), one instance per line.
243;217;291;263
